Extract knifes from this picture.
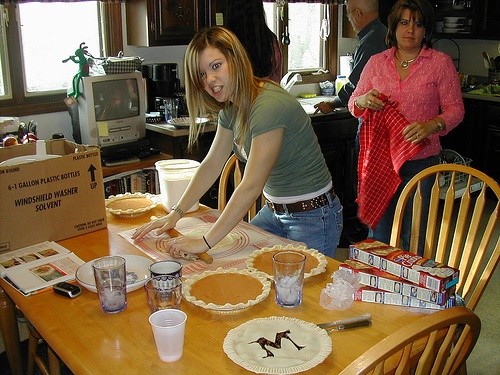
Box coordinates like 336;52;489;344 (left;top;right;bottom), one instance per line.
315;315;370;329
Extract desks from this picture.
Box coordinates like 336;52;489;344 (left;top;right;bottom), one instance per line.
0;193;459;375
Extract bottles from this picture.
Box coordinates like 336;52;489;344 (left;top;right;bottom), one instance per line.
335;75;348;96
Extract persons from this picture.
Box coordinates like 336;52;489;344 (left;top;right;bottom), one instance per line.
312;0;388;243
224;0;282;84
348;0;465;257
131;26;342;260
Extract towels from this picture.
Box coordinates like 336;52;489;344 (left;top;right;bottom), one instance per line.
355;92;432;230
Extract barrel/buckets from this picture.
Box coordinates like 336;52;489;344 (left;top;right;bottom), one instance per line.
154;159;200;214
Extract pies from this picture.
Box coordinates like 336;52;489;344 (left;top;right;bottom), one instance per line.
186;272;263;305
252;250;319;275
106;197;154;211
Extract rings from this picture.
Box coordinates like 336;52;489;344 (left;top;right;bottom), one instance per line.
415;133;419;138
174;240;177;244
368;100;372;105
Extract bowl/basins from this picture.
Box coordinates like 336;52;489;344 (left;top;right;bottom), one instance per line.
444;16;465;24
321;88;334;96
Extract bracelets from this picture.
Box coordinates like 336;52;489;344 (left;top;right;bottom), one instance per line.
172;205;184;218
203;236;211;249
430;119;442;135
329;100;335;110
355;101;363;108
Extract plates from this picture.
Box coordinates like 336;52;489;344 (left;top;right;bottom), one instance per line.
75;255;155;293
442;23;466;33
245;243;328;282
105;192;160;218
222;316;333;375
181;267;271;314
166;117;210;129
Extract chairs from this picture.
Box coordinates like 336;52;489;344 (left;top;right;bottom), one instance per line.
387;163;500;375
338;306;481;375
218;152;268;224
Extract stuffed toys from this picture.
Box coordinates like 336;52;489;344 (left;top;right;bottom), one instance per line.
62;41;89;99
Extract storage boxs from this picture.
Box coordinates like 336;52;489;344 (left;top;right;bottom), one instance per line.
338;257;457;305
0;137;107;255
348;238;461;293
351;284;455;310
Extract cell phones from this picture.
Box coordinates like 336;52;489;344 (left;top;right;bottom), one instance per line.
53;282;81;298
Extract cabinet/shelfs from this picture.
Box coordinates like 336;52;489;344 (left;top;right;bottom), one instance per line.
102;152;174;198
125;0;500;247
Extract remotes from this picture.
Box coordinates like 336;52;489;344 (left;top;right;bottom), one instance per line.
106;158;140;166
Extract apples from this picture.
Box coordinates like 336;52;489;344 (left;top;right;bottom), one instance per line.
3;133;39;146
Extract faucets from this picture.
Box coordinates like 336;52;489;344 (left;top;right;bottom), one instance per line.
279;71;302;92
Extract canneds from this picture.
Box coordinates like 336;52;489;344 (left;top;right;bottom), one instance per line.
148;260;182;307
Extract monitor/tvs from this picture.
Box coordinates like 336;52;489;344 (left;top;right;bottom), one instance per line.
76;70;146;147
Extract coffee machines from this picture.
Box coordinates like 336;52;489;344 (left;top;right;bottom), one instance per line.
141;63;179;121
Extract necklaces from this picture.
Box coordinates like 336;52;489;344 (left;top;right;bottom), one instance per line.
395;50;417;70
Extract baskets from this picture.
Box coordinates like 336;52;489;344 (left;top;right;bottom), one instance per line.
444;148;473;186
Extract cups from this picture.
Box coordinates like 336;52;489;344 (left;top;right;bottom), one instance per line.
488;68;500;85
163;98;179;123
149;309;188;363
148;260;183;305
92;256;128;314
272;251;306;309
144;275;182;314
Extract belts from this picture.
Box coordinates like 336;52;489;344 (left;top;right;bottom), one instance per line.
266;189;335;213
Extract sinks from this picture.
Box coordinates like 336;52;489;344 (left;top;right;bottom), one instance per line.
292;97;349;117
